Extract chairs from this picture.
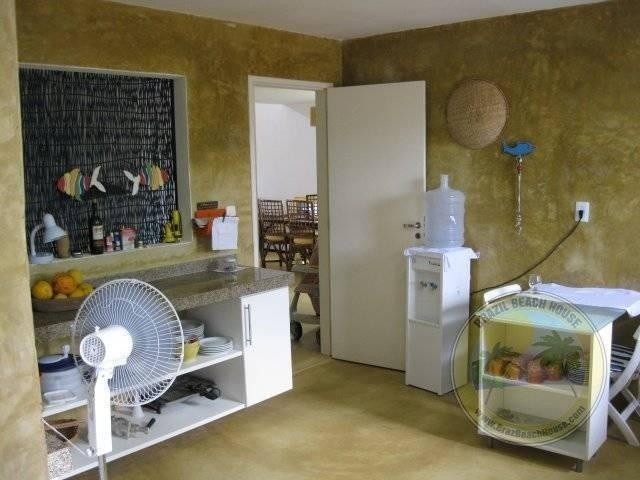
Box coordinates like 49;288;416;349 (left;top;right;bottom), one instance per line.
482;283;522;305
258;193;318;270
607;325;640;446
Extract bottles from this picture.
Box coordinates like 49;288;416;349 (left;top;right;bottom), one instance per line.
164;210;182;244
90;199;105;255
106;226;146;252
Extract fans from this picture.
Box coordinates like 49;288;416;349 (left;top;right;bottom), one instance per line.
71;278;185;480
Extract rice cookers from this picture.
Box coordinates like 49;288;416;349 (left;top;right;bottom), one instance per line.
38;353;84;393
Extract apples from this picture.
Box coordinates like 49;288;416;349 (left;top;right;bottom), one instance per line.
489;350;565;385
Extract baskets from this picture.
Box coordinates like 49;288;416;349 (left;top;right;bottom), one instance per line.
444;78;512;151
32;297;81;312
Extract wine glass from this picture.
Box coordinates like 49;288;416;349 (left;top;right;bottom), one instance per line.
528;274;542;297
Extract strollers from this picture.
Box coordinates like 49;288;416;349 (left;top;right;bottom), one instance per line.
290;237;320;345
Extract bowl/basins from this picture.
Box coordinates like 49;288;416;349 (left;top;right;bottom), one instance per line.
32;294;95;313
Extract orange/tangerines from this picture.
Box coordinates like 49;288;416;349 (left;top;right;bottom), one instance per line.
32;269;93;301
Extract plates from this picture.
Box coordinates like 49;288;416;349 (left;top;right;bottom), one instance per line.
564;352;589;386
169;319;204;343
47;419;81;445
198;336;233;356
42;390;77;408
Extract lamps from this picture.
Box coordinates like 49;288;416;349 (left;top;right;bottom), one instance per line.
28;213;67;263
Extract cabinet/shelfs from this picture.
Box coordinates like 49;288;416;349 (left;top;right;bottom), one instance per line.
185;285;296;409
43;350;245;480
473;283;640;474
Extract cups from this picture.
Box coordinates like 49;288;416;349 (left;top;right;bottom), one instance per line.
52;231;70;259
225;256;239;273
176;336;200;362
486;349;565;385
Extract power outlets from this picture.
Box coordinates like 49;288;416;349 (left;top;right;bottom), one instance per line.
575;200;589;222
